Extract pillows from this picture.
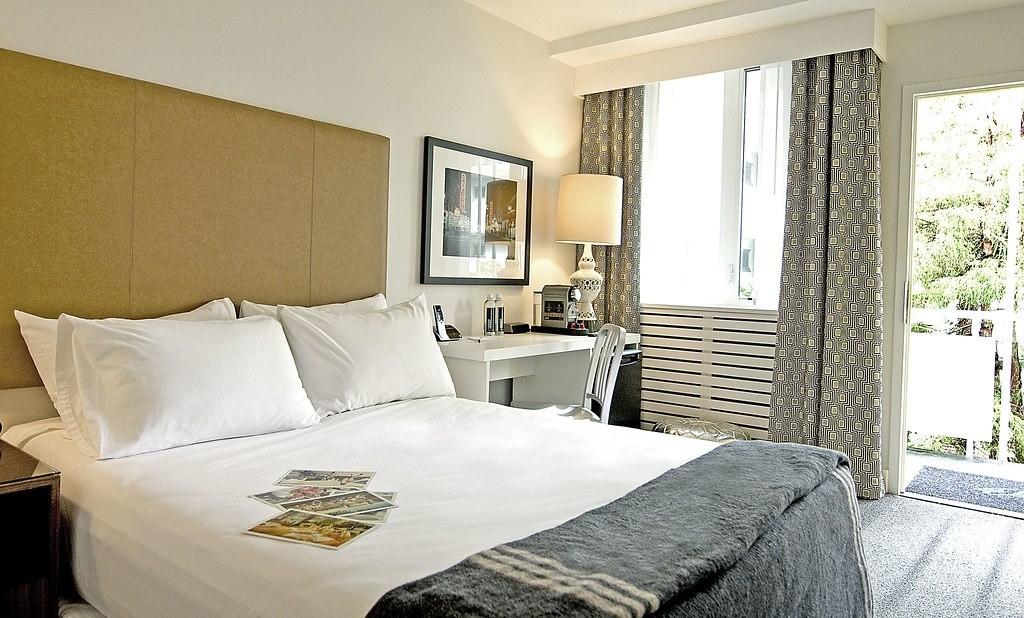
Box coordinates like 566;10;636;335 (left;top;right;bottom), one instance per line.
12;294;457;461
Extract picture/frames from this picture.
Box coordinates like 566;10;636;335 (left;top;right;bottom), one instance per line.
421;136;533;285
555;173;623;334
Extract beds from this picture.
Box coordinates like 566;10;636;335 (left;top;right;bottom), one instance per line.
1;49;874;618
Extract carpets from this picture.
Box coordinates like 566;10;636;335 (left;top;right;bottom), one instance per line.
858;489;1024;618
907;464;1024;516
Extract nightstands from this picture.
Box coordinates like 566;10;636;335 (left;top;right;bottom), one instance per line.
0;436;61;618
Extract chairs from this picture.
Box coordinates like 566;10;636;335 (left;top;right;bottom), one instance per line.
511;324;626;423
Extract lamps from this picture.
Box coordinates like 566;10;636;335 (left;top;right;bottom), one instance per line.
484;180;517;260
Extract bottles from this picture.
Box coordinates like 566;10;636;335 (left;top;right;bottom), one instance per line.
494;293;504;335
483;293;495;336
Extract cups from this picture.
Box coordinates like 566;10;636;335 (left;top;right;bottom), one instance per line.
532;291;542;326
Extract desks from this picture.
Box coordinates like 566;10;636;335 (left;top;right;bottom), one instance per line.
437;332;640;410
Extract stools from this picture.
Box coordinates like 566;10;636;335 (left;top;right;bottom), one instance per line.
652;416;752;445
